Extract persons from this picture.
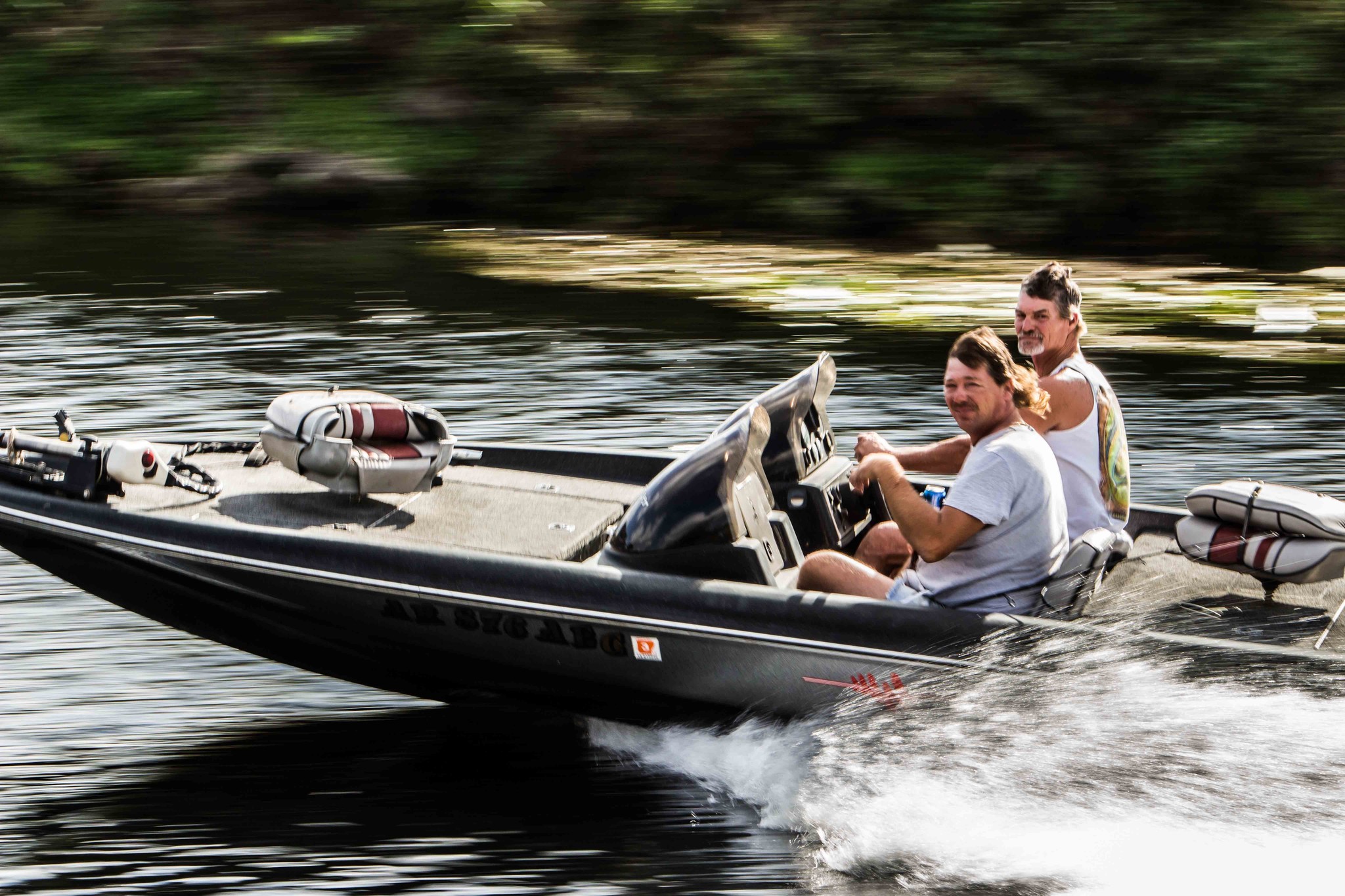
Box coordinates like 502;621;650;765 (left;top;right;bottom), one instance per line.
796;324;1069;617
854;258;1130;549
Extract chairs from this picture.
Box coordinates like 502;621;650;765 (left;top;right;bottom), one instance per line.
1033;528;1132;621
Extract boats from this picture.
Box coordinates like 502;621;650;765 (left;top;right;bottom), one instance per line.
0;352;1345;746
